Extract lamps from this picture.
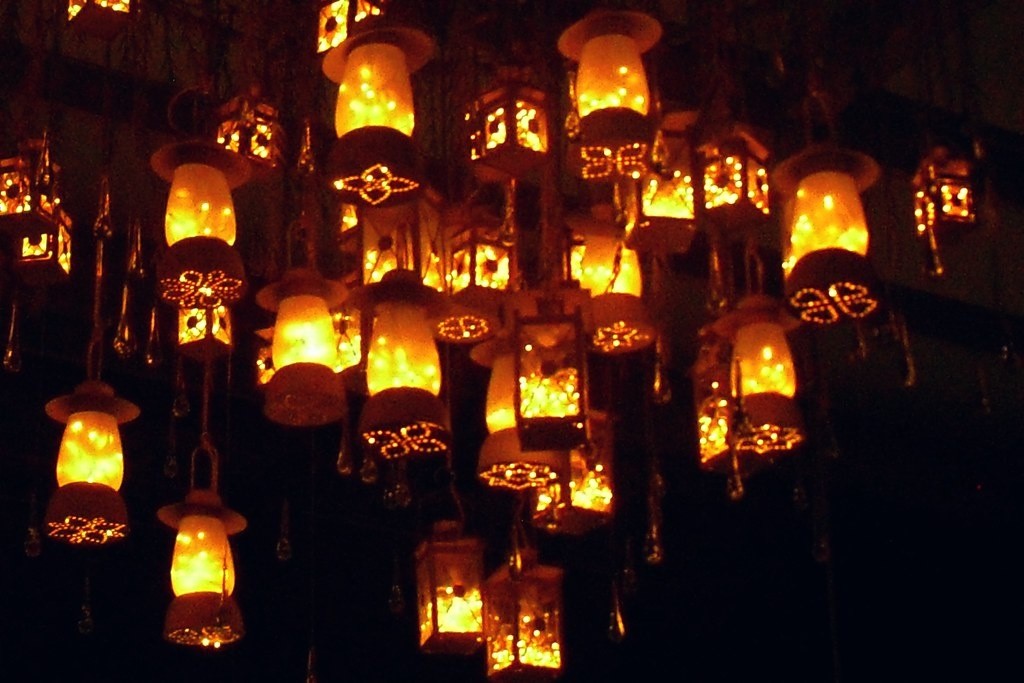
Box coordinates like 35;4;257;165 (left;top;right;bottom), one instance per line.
0;0;987;682
